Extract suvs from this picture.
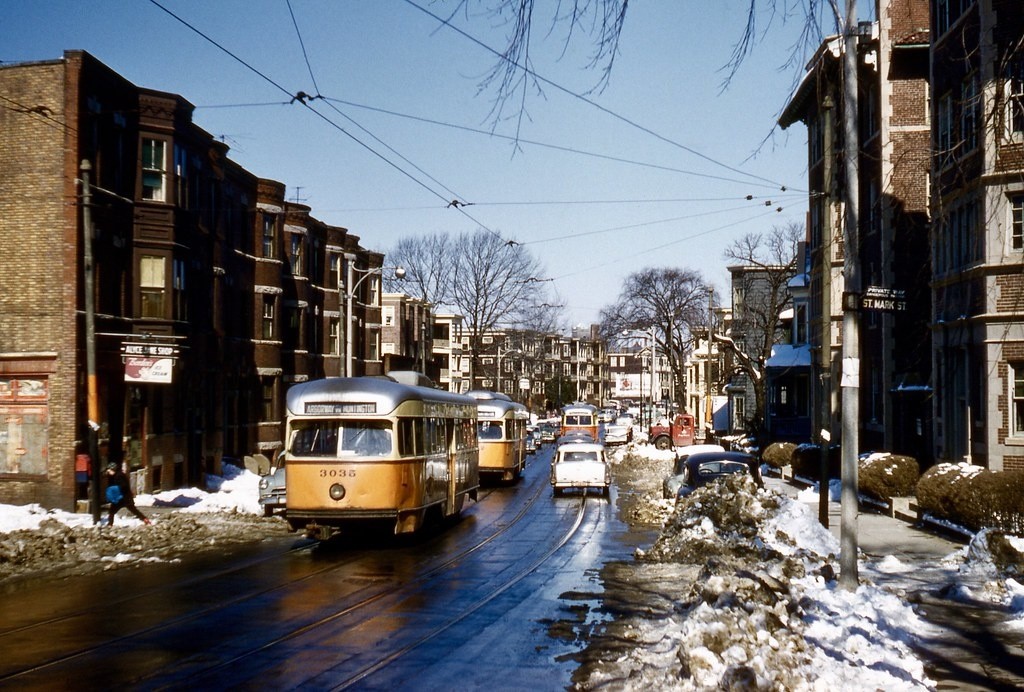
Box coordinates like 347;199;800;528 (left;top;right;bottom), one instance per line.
598;409;617;424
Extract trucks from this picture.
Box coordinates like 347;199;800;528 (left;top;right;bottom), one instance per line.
649;395;728;450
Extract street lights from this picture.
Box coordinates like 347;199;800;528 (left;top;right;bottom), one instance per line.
622;329;656;403
497;348;523;391
347;265;407;377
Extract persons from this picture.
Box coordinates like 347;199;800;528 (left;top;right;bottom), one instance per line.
102;463;152;529
75;446;92;499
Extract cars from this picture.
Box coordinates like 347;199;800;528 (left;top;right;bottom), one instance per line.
259;450;286;516
524;431;536;454
610;399;678;426
605;425;629;444
664;443;727;499
551;444;612;495
422;452;471;487
537;422;555;443
529;426;543;449
553;436;597;460
565;431;592;436
675;450;766;506
552;423;561;437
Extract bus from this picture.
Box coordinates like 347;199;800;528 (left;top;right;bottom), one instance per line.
464;390;527;485
285;370;479;546
560;406;599;444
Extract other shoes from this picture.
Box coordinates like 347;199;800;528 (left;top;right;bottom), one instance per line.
144;518;151;525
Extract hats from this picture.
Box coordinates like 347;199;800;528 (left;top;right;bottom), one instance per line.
105;462;116;469
